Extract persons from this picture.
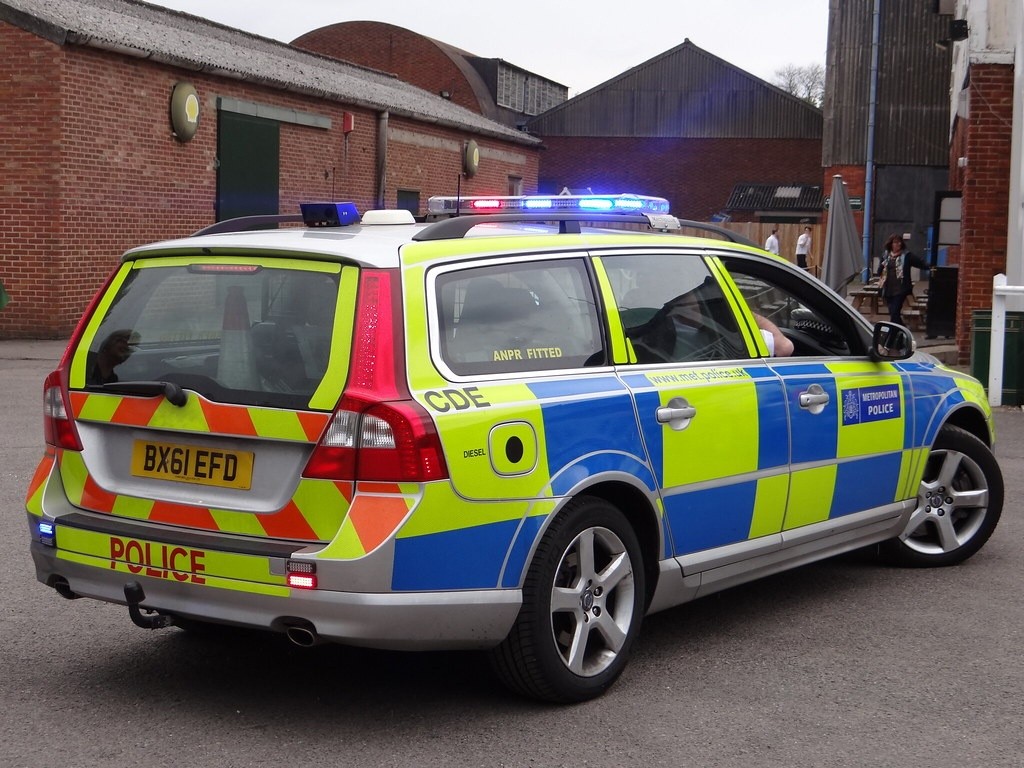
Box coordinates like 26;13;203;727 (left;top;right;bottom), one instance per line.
86;329;140;387
874;233;935;327
764;228;780;256
638;262;794;364
795;227;811;272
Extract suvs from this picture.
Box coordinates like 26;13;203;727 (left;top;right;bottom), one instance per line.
26;189;1006;708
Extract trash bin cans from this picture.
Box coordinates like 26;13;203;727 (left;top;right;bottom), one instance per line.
972;309;1024;406
925;266;959;338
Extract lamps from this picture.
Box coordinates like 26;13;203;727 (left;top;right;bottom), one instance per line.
934;36;954;50
439;90;450;99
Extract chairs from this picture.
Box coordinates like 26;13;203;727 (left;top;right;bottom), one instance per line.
622;287;675;362
455;277;537;325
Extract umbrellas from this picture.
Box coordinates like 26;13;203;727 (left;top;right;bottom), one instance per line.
820;174;864;300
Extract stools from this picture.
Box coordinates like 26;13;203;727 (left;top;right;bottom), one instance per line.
903;310;922;332
909;303;927;315
917;298;928;303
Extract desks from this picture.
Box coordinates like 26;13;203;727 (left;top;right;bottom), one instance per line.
863;285;883;296
850;291;880;324
